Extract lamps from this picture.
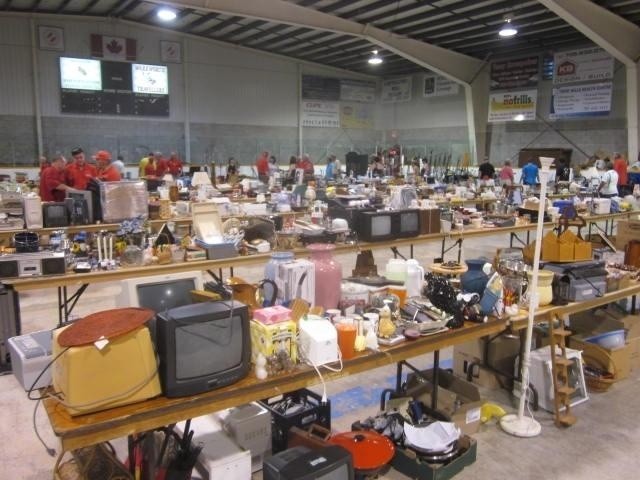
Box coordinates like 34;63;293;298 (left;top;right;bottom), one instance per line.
157;4;177;21
367;50;383;64
499;15;517;37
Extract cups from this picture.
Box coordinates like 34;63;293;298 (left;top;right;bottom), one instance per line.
331;316;357;361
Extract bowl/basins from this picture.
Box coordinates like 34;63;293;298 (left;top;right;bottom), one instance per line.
582;329;625;350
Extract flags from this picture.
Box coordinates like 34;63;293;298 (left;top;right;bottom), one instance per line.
90;33;138;62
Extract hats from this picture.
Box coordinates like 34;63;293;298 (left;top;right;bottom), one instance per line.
72;147;84;155
92;150;111;160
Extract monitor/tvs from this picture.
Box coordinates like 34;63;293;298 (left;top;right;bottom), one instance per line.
116;270;204;313
327;198;422;243
42;202;70;227
264;443;354;480
155;299;252;399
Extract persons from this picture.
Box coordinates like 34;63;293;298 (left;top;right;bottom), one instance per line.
257;152;279;182
324;154;341;179
478;156;495;181
290;152;314;178
520;157;541;186
137;149;183;178
551;152;628;196
36;150;125;201
366;152;431;180
227;155;239;183
499;158;515;187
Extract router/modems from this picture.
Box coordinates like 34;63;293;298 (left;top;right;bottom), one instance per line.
96;236;117;267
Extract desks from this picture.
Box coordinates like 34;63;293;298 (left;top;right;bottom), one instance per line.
0;178;640;480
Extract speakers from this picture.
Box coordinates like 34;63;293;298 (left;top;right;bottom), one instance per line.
0;252;68;277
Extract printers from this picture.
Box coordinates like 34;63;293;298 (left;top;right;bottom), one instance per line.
541;259;609;301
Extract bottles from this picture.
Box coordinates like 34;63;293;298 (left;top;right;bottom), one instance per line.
387;259;424;297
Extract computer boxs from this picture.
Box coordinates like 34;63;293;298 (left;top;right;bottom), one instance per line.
22;192;43;230
213;401;273;473
174;413;252;480
